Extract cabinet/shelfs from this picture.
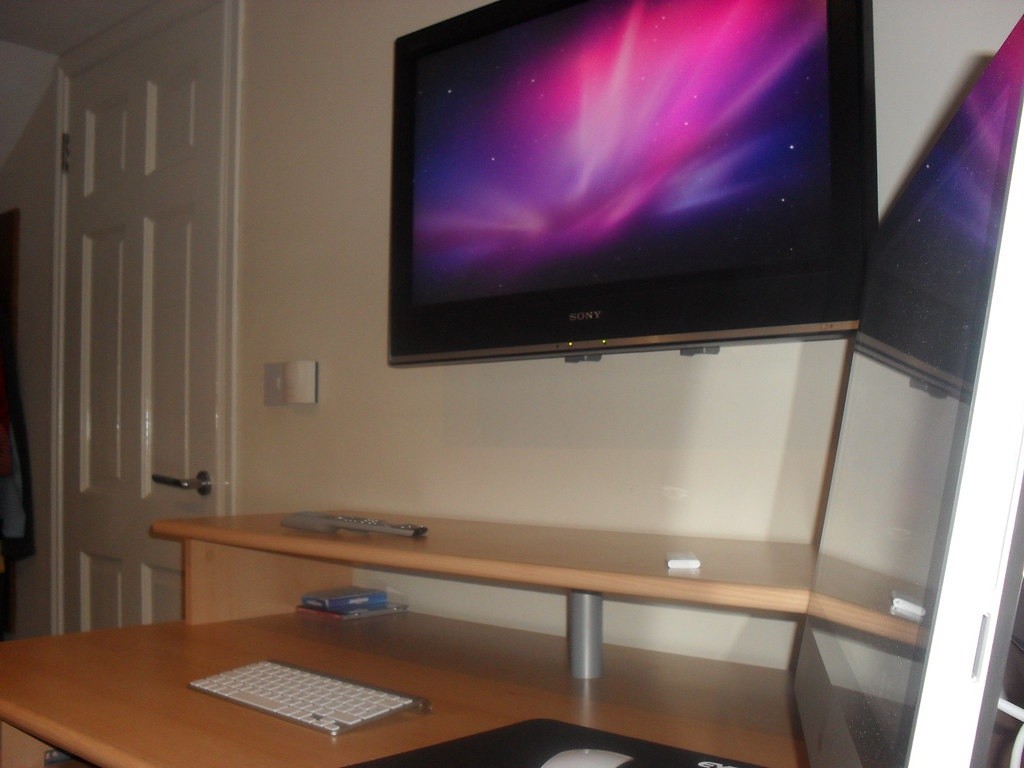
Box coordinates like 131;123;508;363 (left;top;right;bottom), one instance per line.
811;548;946;650
2;505;815;767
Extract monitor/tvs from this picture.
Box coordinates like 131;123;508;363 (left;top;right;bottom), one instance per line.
783;17;1024;767
388;1;881;370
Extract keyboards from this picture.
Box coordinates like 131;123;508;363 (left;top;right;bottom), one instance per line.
187;656;430;735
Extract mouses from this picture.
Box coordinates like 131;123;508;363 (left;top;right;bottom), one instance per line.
540;745;633;767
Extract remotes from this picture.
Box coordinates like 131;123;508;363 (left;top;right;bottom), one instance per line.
277;509;428;539
